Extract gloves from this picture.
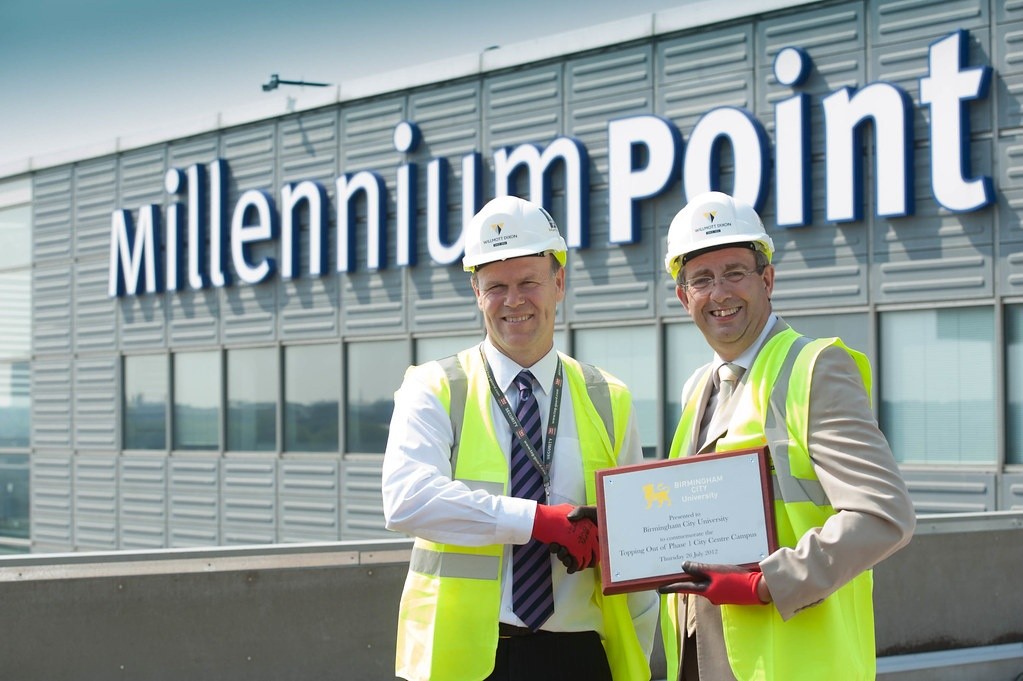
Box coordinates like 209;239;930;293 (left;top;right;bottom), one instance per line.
658;560;768;606
548;504;600;574
532;503;601;575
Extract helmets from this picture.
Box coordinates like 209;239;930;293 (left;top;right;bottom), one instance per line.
665;191;775;272
463;196;568;272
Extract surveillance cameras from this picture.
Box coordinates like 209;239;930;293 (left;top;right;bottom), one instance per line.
262;79;277;91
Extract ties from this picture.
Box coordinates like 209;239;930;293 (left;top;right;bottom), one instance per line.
685;363;744;637
510;369;556;633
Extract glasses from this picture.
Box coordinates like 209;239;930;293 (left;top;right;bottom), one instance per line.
681;263;766;296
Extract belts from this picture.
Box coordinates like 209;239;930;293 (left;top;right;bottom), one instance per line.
499;621;552;639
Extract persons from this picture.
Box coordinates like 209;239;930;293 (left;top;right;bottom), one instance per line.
381;195;659;681
550;192;916;681
